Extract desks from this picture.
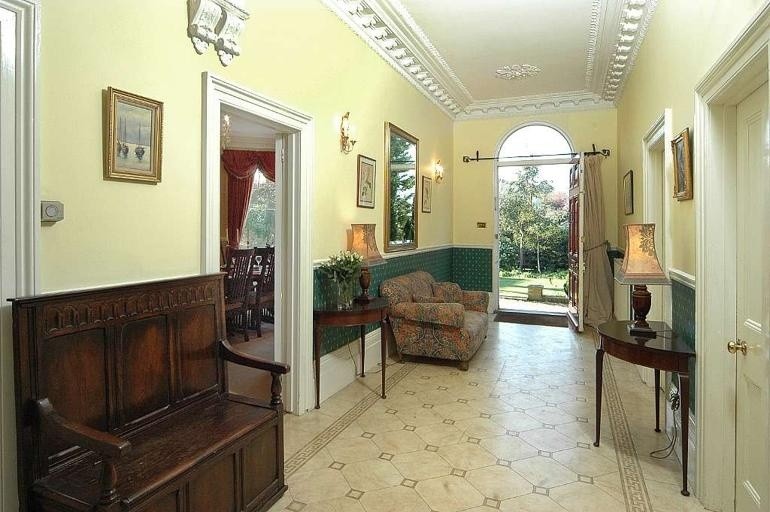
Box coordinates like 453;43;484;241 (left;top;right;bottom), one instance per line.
591;321;694;497
315;296;389;409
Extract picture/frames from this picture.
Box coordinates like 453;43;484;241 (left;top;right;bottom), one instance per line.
671;125;692;201
622;171;634;215
356;154;376;209
422;175;433;213
107;85;163;185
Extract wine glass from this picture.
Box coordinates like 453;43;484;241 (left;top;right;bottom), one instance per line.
255;255;262;268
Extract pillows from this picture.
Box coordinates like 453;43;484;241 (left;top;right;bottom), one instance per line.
413;295;445;303
432;280;464;305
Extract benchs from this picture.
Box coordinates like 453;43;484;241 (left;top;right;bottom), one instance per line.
8;269;292;511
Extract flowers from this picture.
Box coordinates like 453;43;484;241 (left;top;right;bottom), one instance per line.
315;249;364;308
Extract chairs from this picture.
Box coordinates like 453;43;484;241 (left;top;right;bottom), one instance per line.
219;243;274;339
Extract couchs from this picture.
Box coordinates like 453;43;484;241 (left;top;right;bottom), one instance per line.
380;269;491;371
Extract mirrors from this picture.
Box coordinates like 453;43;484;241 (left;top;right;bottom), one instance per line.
383;121;421;252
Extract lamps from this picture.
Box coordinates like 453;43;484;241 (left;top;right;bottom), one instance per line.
614;223;672;336
347;223;388;305
338;109;357;153
435;159;444;183
